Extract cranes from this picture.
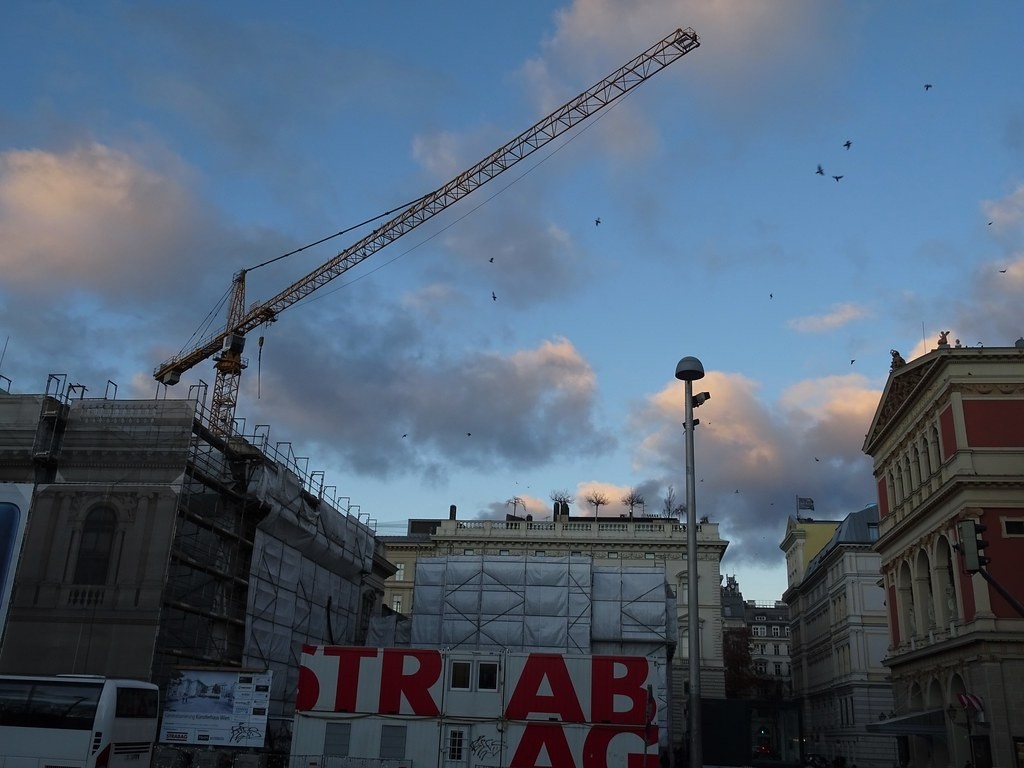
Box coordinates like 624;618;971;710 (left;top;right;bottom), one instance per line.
155;29;699;437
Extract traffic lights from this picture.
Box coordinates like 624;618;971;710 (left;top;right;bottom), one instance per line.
957;519;991;573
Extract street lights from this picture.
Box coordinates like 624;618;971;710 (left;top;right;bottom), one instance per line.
677;357;710;768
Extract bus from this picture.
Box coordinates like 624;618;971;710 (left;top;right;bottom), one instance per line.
0;675;160;768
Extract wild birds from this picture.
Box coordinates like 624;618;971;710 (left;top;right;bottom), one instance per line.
402;83;1008;506
